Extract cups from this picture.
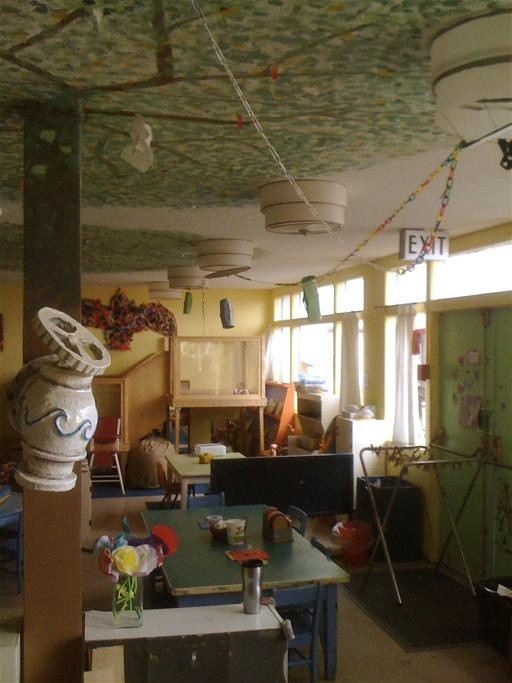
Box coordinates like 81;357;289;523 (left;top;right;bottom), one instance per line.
227;518;245;545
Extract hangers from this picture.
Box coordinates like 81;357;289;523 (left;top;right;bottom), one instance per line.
359;445;481;605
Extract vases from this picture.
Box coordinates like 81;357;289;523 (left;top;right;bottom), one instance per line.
113;577;144;628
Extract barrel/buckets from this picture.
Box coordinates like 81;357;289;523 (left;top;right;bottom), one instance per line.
338;521;372;567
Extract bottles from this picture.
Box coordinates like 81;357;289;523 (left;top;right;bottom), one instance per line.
241;558;263;614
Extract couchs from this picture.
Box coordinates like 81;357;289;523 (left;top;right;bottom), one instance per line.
206;453;353;511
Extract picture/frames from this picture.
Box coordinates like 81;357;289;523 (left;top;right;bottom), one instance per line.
141;408;190;453
211;418;240;449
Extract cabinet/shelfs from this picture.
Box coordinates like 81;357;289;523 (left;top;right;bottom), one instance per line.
234;380;295;445
297;388;339;436
168;335;267;454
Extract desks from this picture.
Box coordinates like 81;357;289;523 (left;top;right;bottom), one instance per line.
165;452;246;510
0;489;23;592
84;602;289;683
142;504;350;683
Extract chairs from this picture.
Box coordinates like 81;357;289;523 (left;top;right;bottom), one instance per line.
287;504;307;535
273;581;322;683
122;516;167;595
188;492;224;510
156;461;195;508
90;416;126;496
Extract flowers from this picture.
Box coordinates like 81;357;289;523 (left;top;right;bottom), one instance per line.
92;525;180;620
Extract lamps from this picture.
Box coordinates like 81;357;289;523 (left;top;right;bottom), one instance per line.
168;266;209;290
148;281;182;300
195;238;254;272
427;10;512;147
259;180;346;235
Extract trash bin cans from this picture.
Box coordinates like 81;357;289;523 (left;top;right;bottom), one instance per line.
473;578;512;662
331;522;372;567
356;476;418;563
